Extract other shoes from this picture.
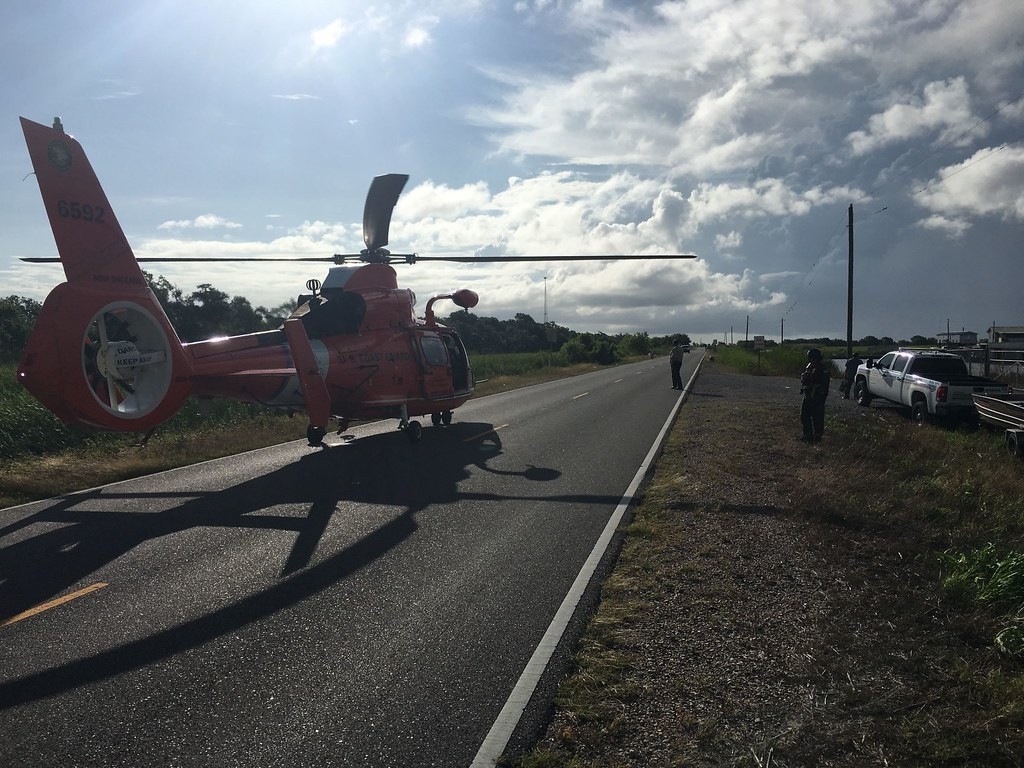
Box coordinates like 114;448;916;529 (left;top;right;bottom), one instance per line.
674;386;682;389
670;385;675;388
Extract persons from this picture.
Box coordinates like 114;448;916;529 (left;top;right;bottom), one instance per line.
798;348;830;445
843;351;863;399
669;339;684;390
650;346;655;359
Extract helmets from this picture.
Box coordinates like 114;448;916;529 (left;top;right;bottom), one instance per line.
852;352;858;355
806;349;821;357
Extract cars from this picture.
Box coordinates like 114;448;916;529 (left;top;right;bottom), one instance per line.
681;345;690;353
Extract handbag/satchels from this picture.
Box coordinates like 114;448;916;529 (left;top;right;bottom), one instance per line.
839;381;846;392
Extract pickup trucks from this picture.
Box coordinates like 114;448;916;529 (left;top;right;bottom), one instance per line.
853;346;1012;427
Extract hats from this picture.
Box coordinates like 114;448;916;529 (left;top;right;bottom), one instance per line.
672;338;679;342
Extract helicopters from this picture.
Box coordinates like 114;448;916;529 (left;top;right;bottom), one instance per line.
12;117;703;454
